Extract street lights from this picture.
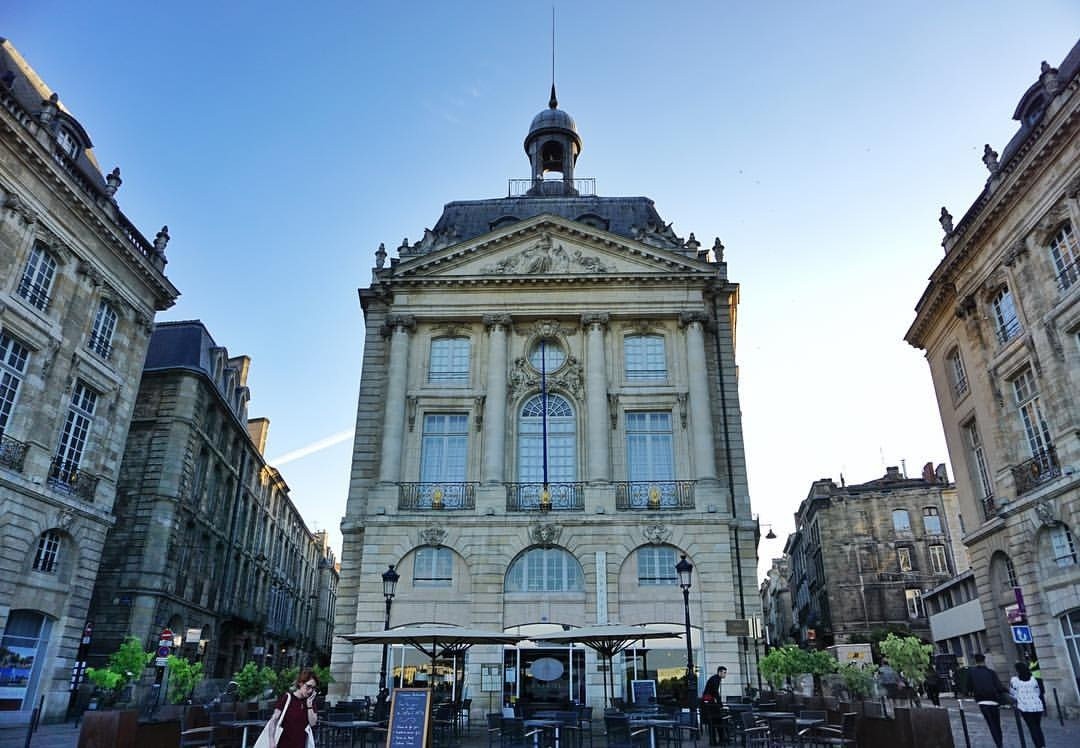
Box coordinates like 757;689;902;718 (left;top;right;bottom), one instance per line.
675;555;700;742
372;564;401;735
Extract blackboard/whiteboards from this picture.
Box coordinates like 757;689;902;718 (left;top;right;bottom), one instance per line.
632;679;656;702
387;688;430;748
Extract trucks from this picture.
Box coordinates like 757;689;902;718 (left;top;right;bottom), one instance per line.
826;644;874;680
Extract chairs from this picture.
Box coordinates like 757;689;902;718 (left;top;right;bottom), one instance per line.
312;696;472;748
603;694;858;748
486;697;594;748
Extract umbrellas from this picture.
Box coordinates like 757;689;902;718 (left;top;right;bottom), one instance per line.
336;626;688;704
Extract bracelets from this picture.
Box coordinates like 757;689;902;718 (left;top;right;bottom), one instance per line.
306;706;313;709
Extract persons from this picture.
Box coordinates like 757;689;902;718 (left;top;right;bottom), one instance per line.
702;665;733;745
269;670;319;748
877;654;1047;748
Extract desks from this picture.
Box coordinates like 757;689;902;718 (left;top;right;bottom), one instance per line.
798;718;825;748
325;720;377;748
630;719;681;747
218;720;268;748
755;712;797;748
522;720;564;748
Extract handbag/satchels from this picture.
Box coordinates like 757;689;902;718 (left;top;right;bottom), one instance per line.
253;718;283;748
305;723;315;748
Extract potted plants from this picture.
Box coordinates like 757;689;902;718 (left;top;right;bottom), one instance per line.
222;662;278;721
840;662;881;720
878;631;955;748
155;655;209;740
76;635;150;748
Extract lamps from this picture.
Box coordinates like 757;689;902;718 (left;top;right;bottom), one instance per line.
759;524;776;538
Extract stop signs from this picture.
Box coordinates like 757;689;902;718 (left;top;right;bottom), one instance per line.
161;631;172;640
